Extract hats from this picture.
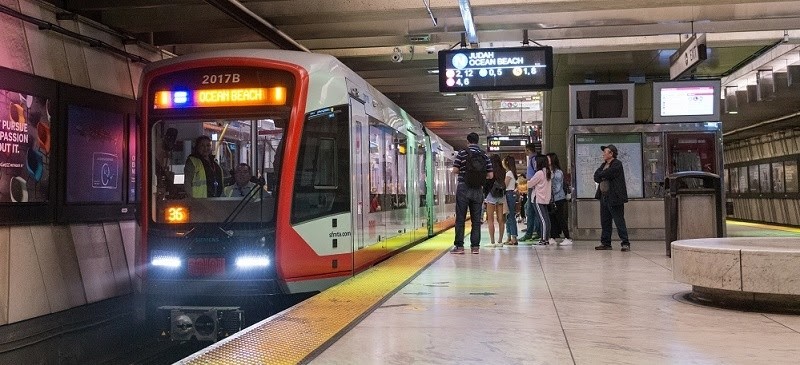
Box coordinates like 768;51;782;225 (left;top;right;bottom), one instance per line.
601;145;619;154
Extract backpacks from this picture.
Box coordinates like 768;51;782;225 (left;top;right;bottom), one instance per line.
490;180;507;199
462;146;487;190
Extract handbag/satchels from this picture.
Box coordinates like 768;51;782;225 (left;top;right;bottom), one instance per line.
547;202;558;214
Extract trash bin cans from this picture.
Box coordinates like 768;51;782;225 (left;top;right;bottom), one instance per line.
663;171;724;258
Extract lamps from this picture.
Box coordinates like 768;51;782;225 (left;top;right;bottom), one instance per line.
391;48;403;63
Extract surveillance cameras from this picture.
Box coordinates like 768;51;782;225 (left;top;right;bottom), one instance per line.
392;52;404;63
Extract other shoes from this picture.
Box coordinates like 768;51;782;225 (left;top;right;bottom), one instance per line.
503;241;518;245
530;235;541;241
495;242;503;247
471;246;480;253
559;238;573;246
595;244;612;250
450;246;464;254
532;241;549;246
621;245;630;252
484;243;496;246
548;238;557;246
518;235;532;242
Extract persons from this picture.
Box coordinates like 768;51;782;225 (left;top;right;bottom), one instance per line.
183;135;223;199
450;133;494;254
483;154;572;248
593;144;631;251
219;162;272;199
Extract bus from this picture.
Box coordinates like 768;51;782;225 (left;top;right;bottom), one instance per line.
135;48;471;342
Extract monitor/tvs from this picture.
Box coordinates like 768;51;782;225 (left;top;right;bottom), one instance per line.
569;83;634;125
654;80;720;124
